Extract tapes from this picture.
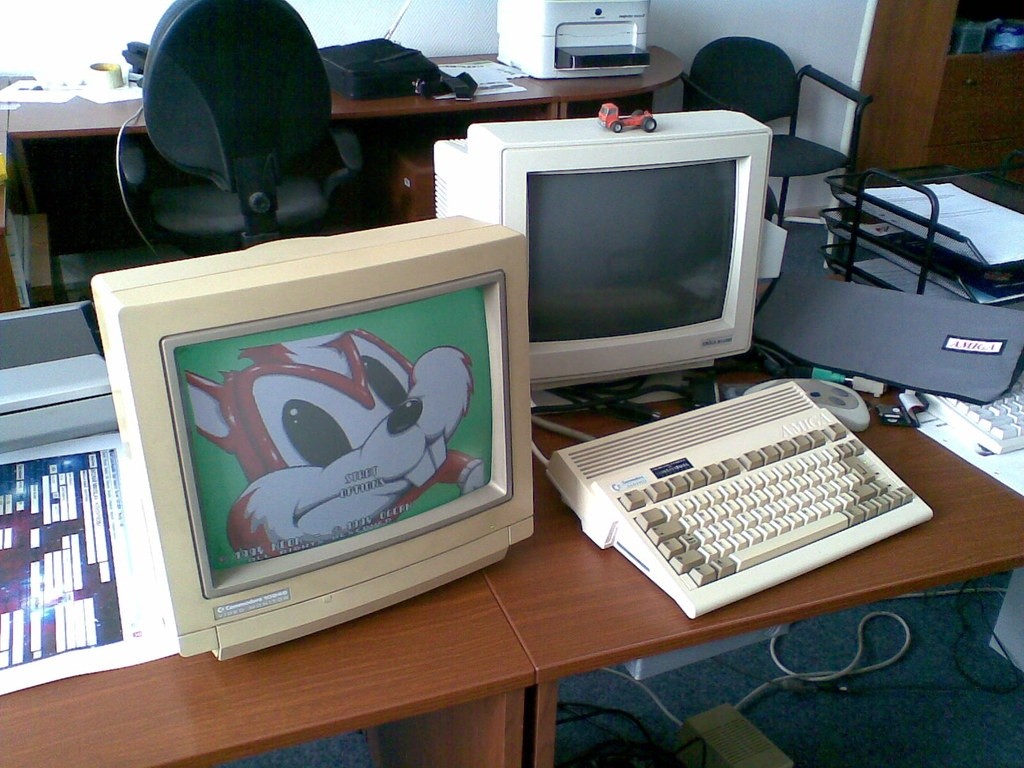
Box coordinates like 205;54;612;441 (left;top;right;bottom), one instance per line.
89;61;123;89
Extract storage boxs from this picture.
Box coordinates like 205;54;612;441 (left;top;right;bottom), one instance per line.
55;247;193;303
28;214;53;308
380;128;462;222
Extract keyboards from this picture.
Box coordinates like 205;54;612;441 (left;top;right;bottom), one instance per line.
545;381;933;619
921;370;1024;455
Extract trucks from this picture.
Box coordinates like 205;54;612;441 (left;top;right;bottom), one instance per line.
596;102;657;133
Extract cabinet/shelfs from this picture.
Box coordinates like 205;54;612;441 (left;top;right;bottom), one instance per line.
857;0;1023;181
817;150;1023;312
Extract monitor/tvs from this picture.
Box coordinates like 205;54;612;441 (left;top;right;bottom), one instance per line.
435;108;774;410
93;216;534;664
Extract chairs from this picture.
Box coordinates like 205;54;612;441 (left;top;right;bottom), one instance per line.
680;36;874;254
118;0;365;261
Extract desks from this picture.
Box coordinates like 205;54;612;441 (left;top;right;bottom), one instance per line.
8;54;557;310
485;352;1024;768
498;45;683;120
0;566;535;768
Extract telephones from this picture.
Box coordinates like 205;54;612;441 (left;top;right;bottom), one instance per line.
122;42;150;75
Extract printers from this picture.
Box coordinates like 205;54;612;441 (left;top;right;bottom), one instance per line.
496;0;652;80
